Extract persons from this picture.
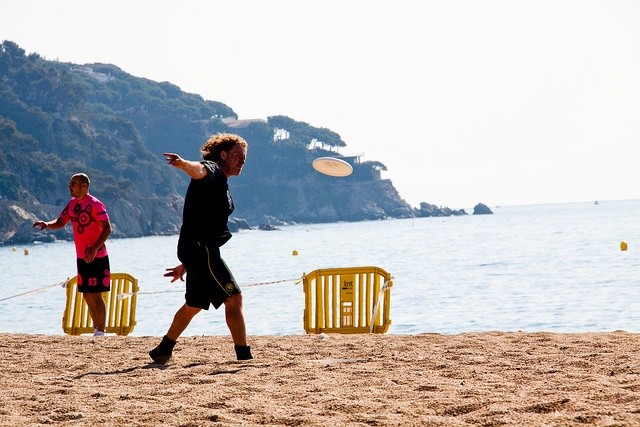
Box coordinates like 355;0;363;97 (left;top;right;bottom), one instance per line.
147;134;254;365
33;172;112;337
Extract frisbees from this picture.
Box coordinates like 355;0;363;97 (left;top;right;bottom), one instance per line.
312;157;353;177
